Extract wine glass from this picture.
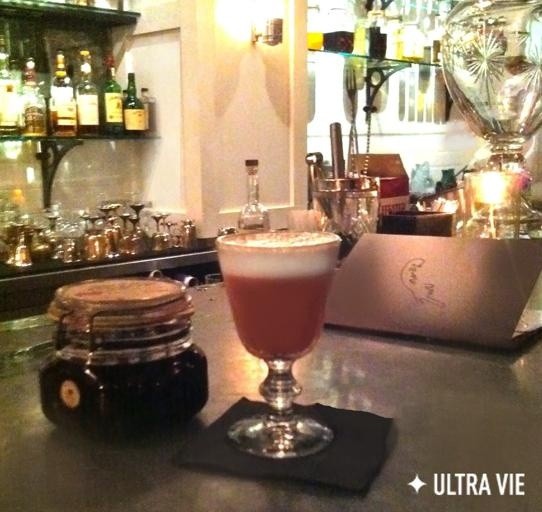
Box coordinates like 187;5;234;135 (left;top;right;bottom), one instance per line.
213;229;344;463
0;200;197;274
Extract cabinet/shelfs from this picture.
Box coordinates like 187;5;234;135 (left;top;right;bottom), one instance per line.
0;1;159;143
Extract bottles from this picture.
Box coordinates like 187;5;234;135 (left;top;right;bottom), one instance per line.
36;277;210;444
138;87;156;137
123;72;149;136
100;56;124;137
307;3;428;63
77;50;103;136
236;159;271;231
1;34;47;137
47;49;78;137
